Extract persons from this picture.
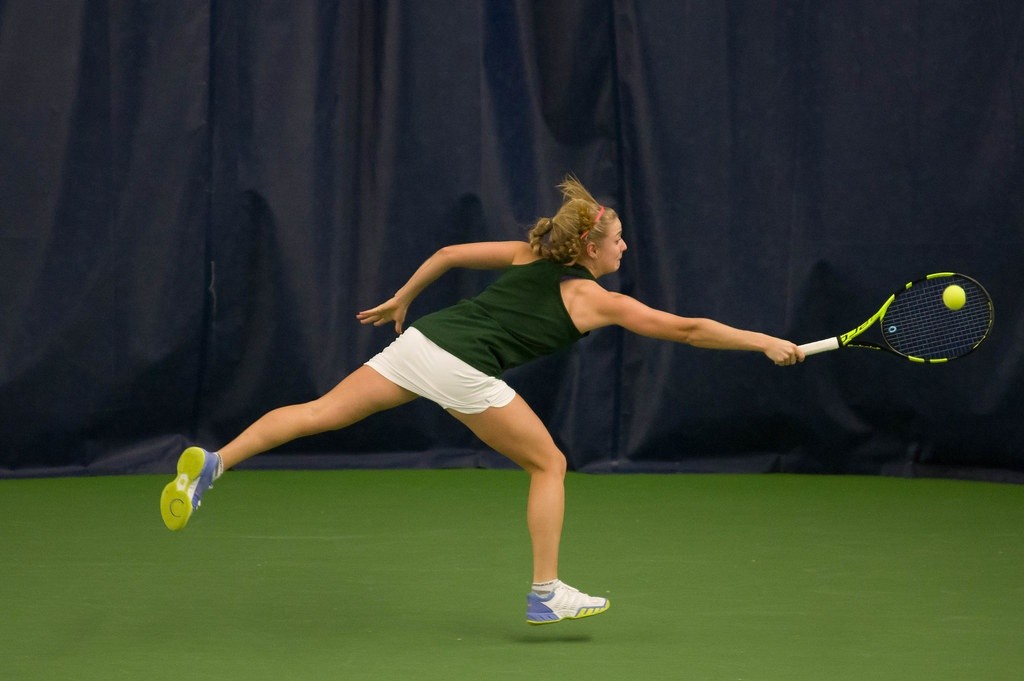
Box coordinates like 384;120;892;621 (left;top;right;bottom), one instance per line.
160;180;806;623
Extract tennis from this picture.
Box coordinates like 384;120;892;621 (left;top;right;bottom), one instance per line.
940;283;968;312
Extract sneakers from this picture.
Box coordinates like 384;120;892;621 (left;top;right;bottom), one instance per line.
526;581;610;626
160;446;218;532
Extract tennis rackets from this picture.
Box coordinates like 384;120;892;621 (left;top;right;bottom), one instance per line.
774;269;995;363
